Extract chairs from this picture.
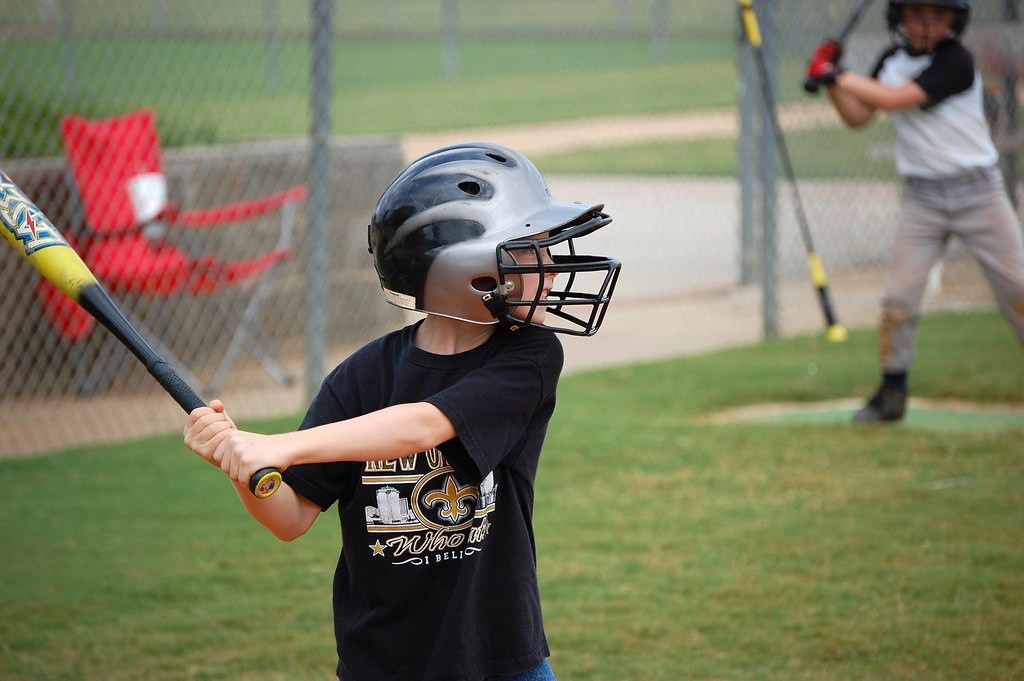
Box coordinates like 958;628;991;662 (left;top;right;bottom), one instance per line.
35;107;308;401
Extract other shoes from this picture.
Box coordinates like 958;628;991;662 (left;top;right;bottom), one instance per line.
854;387;907;422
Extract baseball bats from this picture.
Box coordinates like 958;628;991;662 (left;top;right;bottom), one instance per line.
1;168;283;500
802;0;879;94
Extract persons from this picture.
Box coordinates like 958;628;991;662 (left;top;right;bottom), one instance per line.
182;141;622;681
808;0;1024;424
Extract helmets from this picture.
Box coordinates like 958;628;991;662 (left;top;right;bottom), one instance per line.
888;0;972;40
369;142;621;336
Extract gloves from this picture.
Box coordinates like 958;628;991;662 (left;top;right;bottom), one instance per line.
807;38;843;85
